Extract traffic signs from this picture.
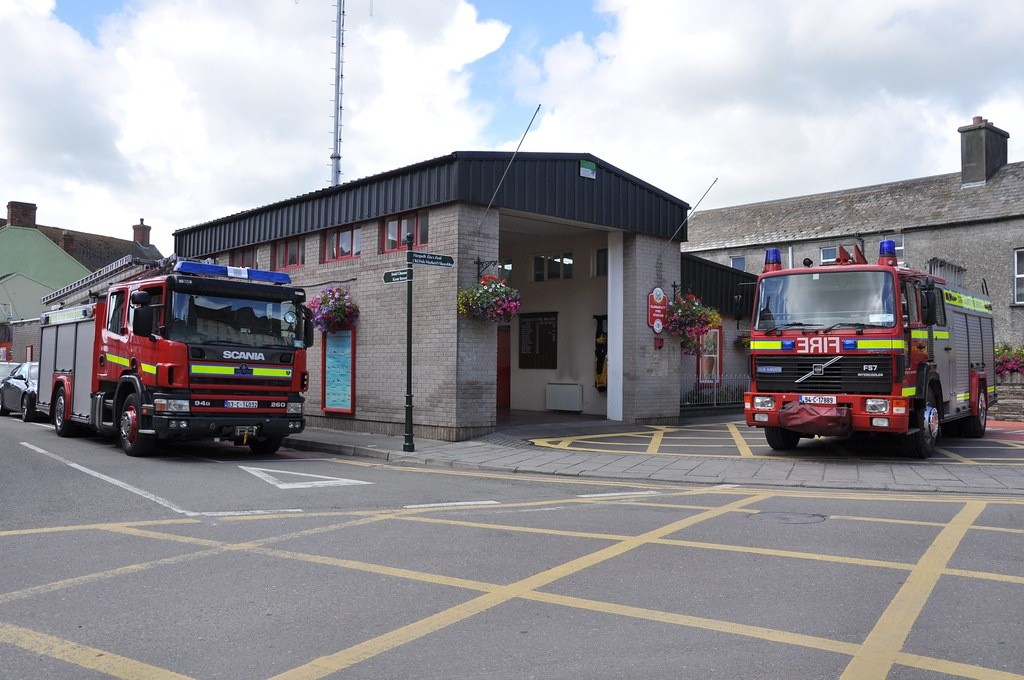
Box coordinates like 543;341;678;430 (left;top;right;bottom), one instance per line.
382;267;413;284
406;250;455;268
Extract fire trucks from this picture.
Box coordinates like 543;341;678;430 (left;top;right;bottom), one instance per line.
742;239;999;460
30;259;315;457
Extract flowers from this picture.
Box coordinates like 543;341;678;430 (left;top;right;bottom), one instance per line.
304;286;359;335
457;274;521;324
994;345;1024;378
664;293;724;359
734;335;750;350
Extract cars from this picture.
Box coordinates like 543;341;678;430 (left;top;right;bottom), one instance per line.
0;362;49;422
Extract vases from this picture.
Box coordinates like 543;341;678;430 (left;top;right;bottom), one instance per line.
996;372;1024;384
